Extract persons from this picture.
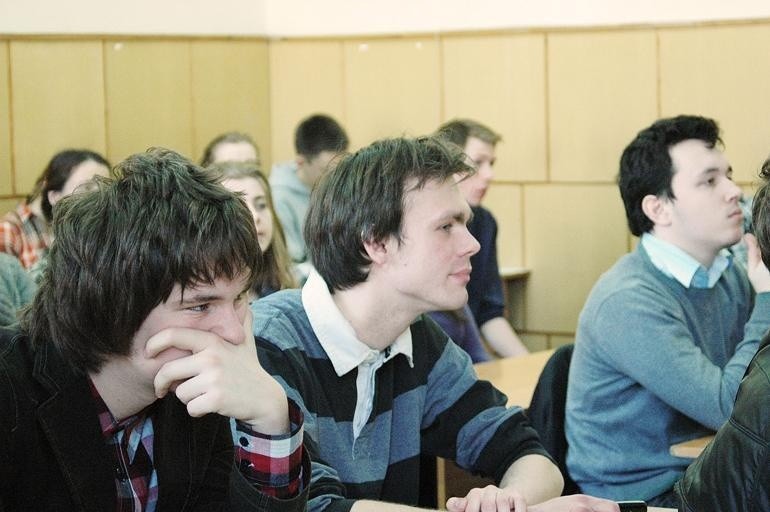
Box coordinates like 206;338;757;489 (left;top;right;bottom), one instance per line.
248;137;622;512
0;148;112;327
416;119;530;363
565;112;769;512
201;116;350;302
1;147;311;512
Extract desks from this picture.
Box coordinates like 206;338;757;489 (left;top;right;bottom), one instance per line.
430;340;558;512
